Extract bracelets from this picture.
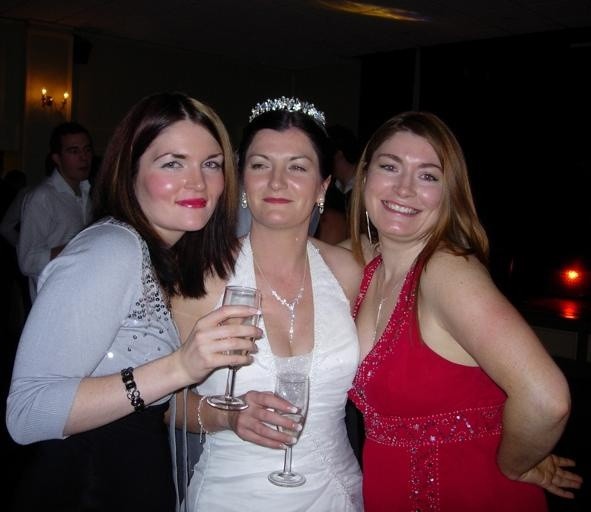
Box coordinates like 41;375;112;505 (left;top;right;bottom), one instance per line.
198;392;219;443
123;365;145;412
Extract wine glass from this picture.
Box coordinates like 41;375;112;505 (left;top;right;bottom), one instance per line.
204;285;263;412
266;371;311;488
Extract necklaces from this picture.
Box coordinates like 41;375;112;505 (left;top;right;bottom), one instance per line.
252;249;309;347
372;259;410;338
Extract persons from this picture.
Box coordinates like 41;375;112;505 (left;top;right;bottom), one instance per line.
2;125;98;306
237;151;358;245
5;79;264;512
152;93;373;512
339;110;586;510
